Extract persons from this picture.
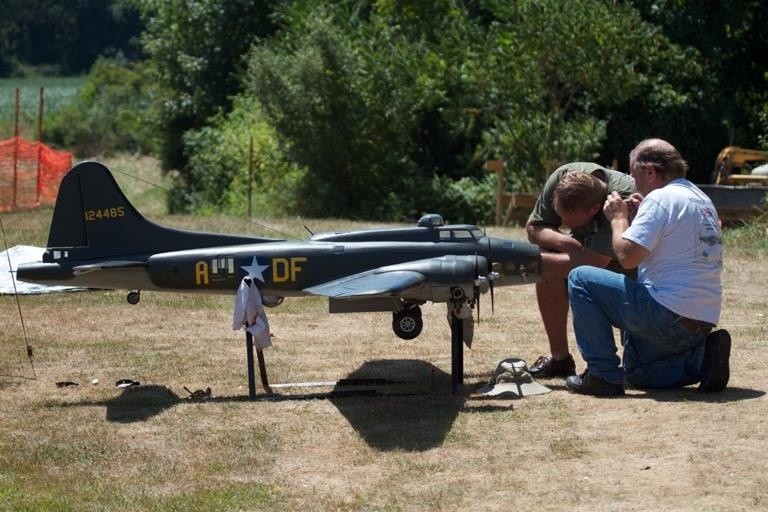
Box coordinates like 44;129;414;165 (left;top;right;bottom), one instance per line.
566;137;732;396
524;161;644;380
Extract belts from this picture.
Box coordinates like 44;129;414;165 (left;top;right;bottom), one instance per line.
676;313;715;330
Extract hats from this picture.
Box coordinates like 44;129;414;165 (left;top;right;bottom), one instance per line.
478;357;553;397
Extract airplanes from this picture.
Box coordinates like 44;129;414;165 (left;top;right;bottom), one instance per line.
0;162;540;340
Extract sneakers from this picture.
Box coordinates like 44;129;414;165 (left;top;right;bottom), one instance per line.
567;370;626;397
697;327;732;393
528;353;577;378
